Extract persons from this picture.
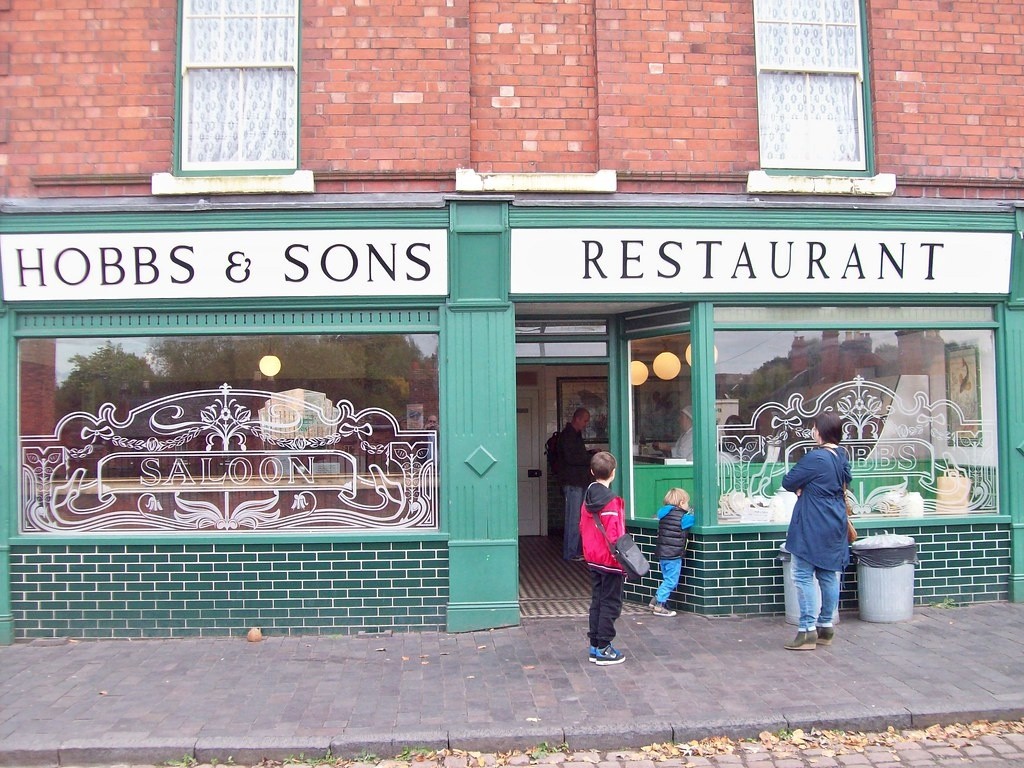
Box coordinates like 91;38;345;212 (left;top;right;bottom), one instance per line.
649;488;695;617
652;406;692;458
556;407;602;561
580;451;627;664
781;411;852;651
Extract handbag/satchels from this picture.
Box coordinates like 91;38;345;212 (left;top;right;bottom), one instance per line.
936;469;971;515
847;519;858;543
615;533;649;579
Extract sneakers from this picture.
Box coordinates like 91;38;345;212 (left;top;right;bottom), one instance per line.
653;602;677;616
589;643;625;665
649;597;658;607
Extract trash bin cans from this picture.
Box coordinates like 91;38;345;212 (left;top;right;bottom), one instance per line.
851;534;916;622
780;541;840;626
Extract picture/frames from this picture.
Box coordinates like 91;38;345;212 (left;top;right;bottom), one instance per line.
556;376;608;444
634;375;692;445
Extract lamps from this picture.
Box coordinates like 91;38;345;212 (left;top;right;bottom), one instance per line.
653;338;681;380
632;346;649;385
259;338;281;376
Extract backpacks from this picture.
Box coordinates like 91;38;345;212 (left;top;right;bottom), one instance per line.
544;431;563;472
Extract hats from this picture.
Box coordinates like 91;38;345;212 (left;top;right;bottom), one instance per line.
681;406;693;419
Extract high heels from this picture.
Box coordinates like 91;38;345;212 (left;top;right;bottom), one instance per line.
783;630;818;649
816;626;834;645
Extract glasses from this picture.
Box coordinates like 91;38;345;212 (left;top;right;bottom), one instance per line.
429;420;436;423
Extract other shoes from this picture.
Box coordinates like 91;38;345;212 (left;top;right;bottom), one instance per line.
570;554;585;561
